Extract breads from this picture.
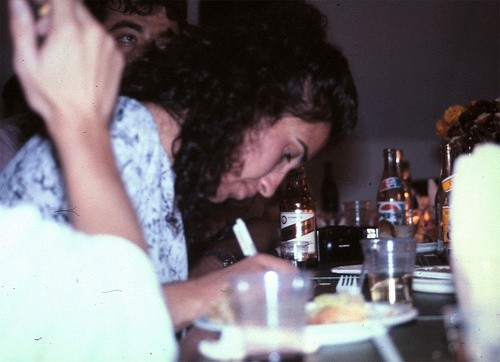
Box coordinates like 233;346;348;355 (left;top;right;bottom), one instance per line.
304;292;369;326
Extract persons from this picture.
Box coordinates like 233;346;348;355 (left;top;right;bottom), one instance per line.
2;0;357;362
1;0;181;362
0;0;188;169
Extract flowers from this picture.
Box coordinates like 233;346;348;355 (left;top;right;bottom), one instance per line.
436;91;500;153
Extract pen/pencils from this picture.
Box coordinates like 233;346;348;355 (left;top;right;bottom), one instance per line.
233;217;257;256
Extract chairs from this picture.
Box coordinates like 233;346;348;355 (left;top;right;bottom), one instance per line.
315;225;375;271
